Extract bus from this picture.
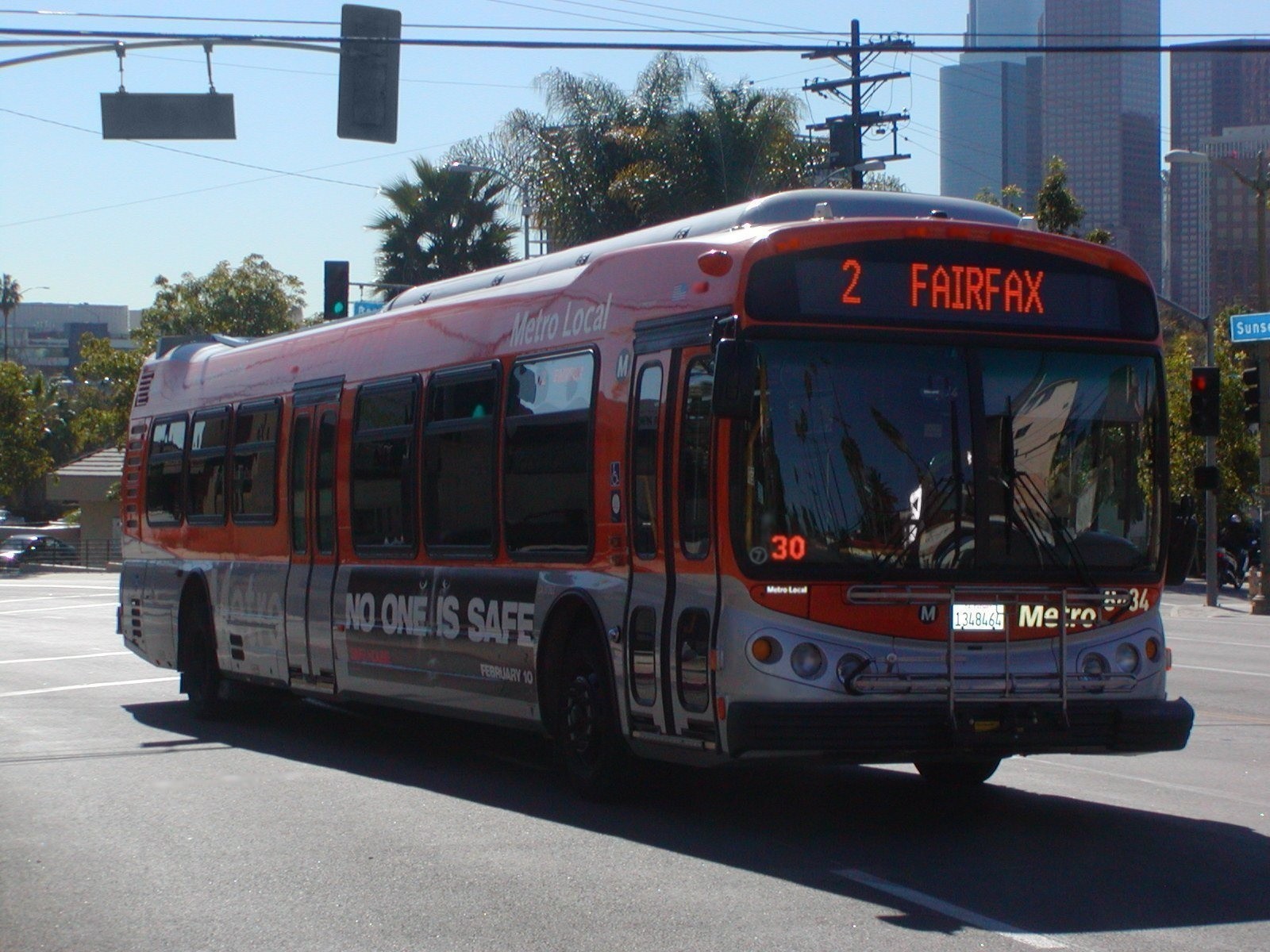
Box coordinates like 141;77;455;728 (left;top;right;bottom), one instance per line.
115;190;1194;790
146;398;708;555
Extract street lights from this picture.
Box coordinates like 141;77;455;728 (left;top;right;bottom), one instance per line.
1165;150;1270;615
451;163;531;260
11;286;50;362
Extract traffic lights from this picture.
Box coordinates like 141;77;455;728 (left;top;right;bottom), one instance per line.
1241;366;1258;424
1192;466;1219;490
323;260;350;321
1189;366;1217;437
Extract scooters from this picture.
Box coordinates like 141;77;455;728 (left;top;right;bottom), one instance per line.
1208;515;1251;590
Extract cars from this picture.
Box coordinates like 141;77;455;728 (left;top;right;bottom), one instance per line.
0;509;13;525
0;535;79;571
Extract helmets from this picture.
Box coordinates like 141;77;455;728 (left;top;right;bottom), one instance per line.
1230;514;1241;523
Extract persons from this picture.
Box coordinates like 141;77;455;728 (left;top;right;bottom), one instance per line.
1218;513;1255;579
